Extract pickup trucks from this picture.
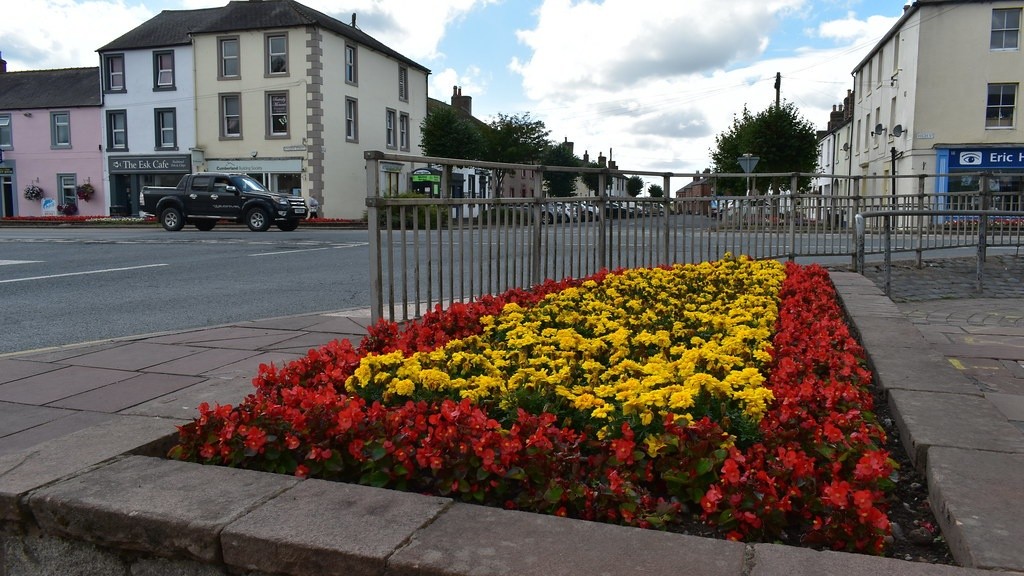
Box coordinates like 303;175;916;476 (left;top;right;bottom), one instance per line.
142;173;309;232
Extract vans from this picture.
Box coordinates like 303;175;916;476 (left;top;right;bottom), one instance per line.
669;201;680;214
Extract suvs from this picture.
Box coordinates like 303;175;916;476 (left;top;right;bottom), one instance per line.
742;199;773;218
645;202;666;217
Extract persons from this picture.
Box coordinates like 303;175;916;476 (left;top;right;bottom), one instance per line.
308;195;321;219
218;180;228;193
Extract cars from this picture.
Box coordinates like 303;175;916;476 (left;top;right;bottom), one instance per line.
708;199;739;218
605;203;648;218
491;200;600;224
